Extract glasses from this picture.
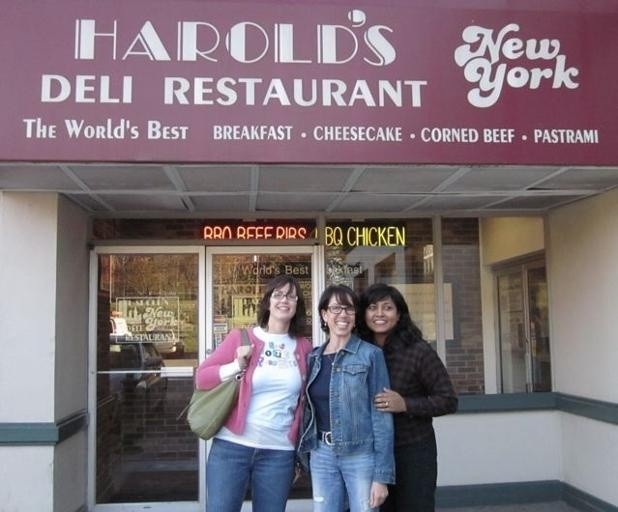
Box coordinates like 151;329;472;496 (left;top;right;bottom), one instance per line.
327;305;356;315
271;291;299;302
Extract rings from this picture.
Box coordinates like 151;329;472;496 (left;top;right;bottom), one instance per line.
385;401;389;407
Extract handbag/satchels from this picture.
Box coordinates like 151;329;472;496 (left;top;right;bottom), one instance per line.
185;326;251;440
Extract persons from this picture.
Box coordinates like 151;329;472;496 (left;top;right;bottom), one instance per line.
193;276;313;511
357;283;458;511
298;283;398;512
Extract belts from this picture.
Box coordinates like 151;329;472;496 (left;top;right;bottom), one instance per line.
316;431;332;446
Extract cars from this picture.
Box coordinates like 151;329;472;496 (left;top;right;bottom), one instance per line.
110;317;184;432
